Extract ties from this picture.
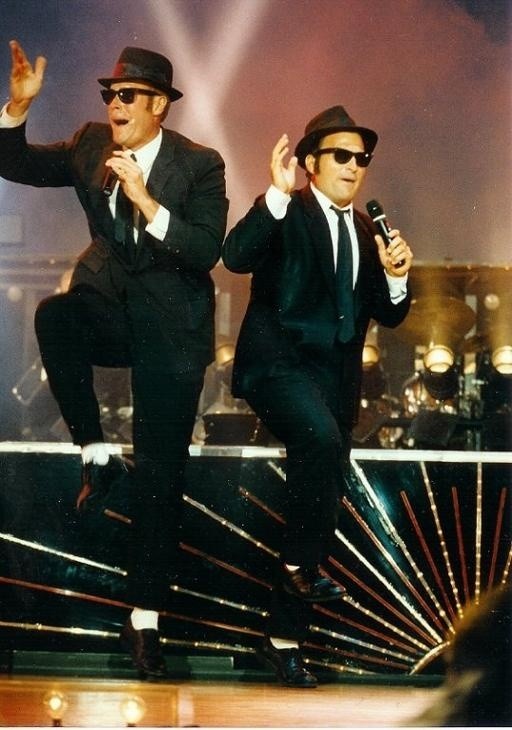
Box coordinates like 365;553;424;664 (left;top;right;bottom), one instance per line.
328;205;358;345
112;153;138;268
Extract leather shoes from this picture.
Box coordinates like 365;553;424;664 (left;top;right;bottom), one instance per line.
77;453;135;518
255;635;320;690
275;561;347;605
115;618;168;680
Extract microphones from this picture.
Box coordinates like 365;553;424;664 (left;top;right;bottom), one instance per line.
102;149;133;198
366;199;406;271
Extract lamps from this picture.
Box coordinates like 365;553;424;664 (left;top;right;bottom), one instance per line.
358;340;512;406
211;332;237;387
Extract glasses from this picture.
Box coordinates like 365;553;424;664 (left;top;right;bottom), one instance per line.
313;146;375;169
99;87;162;106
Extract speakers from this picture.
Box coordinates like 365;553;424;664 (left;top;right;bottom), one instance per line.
198;412;509;451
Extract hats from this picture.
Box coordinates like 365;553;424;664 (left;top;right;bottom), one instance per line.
97;45;184;103
293;105;379;170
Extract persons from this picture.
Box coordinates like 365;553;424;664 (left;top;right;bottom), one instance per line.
221;106;412;688
0;41;229;684
